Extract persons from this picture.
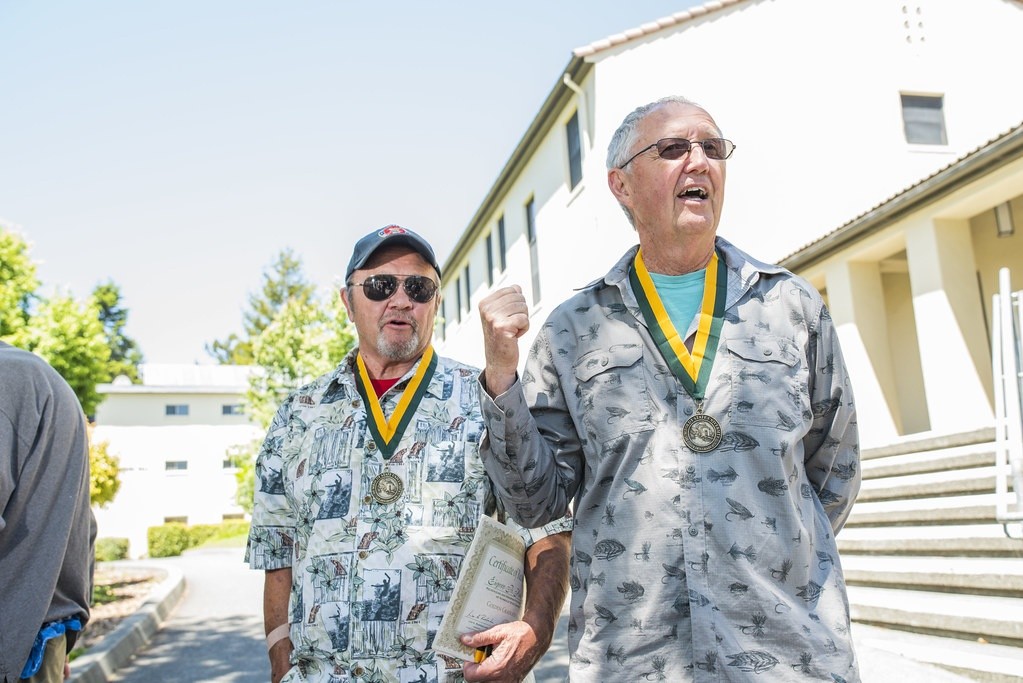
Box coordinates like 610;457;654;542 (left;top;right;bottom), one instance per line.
0;339;98;683
477;98;860;683
243;225;573;683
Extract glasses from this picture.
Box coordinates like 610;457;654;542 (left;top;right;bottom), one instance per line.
349;274;439;303
619;137;737;170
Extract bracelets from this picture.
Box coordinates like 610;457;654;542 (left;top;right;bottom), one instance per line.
266;623;290;652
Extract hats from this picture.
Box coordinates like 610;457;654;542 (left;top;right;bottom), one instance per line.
346;224;441;282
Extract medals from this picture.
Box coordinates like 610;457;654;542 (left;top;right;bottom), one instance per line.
682;414;722;453
371;473;403;504
